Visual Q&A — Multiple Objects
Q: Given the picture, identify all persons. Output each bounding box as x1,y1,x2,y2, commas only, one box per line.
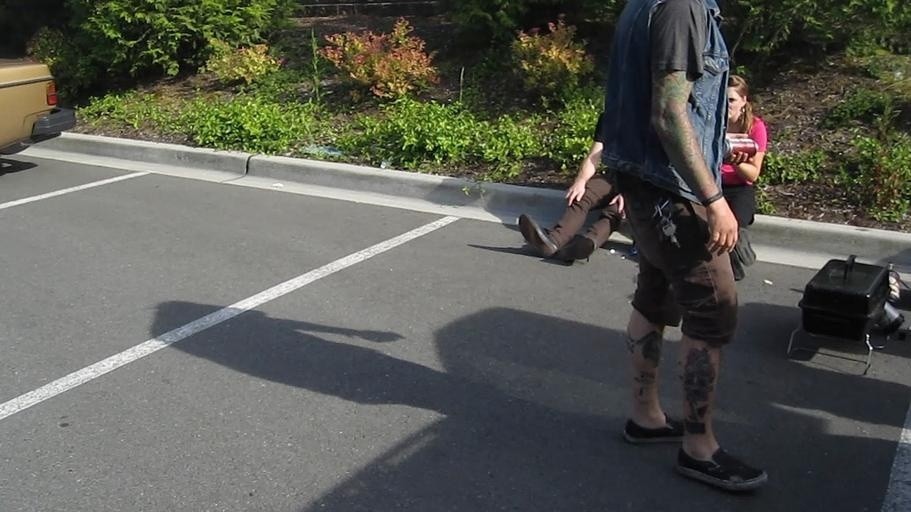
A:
597,0,774,494
518,111,629,264
715,74,771,282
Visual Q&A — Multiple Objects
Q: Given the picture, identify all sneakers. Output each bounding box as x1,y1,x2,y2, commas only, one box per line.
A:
677,446,767,491
518,214,559,259
623,413,683,443
556,235,597,264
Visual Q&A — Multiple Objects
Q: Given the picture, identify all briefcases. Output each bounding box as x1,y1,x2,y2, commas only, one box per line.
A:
798,254,892,340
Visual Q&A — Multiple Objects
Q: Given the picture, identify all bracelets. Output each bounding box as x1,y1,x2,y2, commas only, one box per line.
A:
700,192,725,207
